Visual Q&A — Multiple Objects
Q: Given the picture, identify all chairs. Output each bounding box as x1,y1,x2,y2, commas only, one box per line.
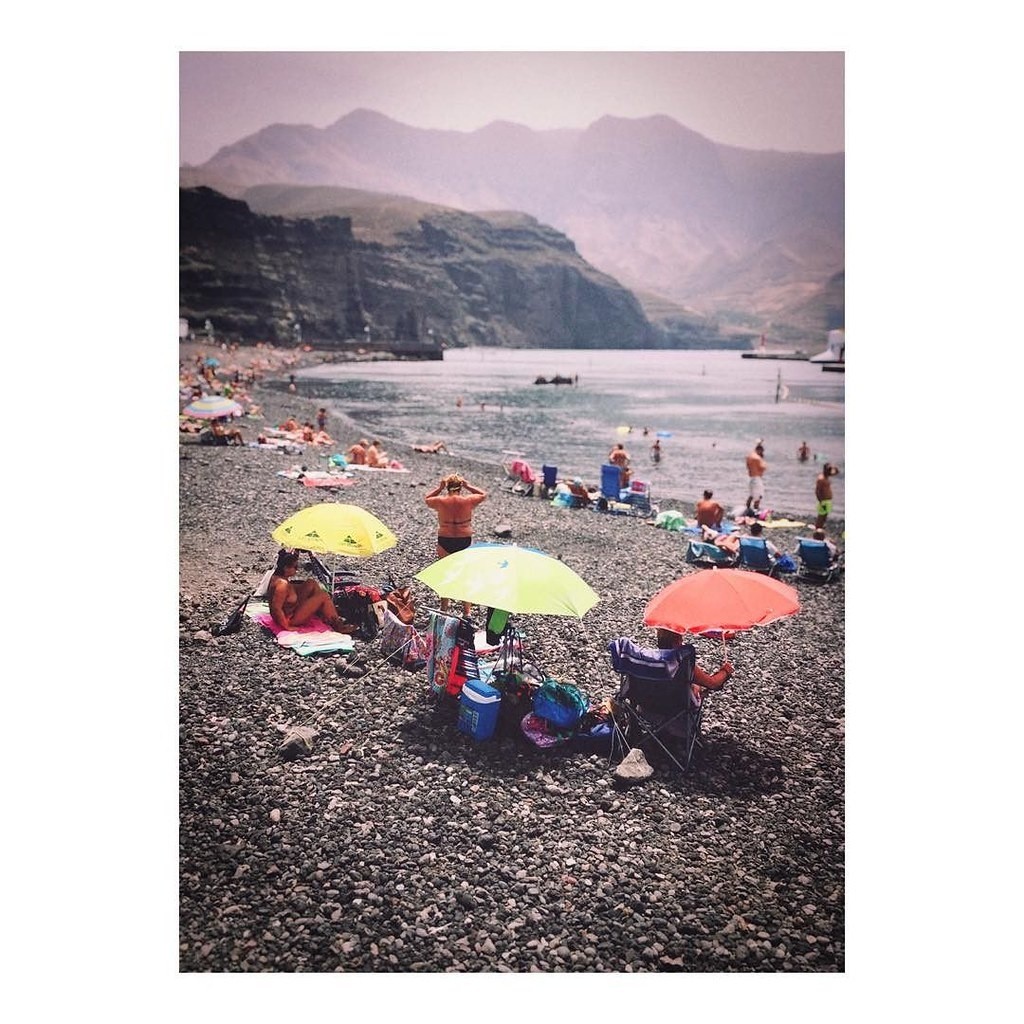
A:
734,535,788,579
495,451,664,520
306,551,360,593
605,633,707,775
794,535,845,583
688,539,734,570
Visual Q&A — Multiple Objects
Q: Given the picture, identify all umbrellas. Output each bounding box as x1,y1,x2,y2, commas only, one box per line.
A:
411,543,602,677
643,565,802,664
183,394,244,430
271,503,398,601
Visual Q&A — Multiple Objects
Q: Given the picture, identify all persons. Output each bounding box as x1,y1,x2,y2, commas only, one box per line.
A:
265,549,339,631
656,627,734,701
179,343,845,584
424,475,488,617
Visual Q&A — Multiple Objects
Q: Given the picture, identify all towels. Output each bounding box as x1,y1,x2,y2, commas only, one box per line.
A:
655,508,689,531
775,554,798,572
683,539,728,563
600,463,623,499
550,483,577,508
328,453,411,473
277,468,360,487
541,464,558,488
631,480,647,492
573,477,584,489
179,352,266,437
247,425,338,455
510,459,537,485
679,507,807,535
228,579,697,755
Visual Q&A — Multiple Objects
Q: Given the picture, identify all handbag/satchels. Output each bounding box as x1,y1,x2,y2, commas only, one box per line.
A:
255,569,277,598
211,595,252,635
384,577,413,624
534,679,591,727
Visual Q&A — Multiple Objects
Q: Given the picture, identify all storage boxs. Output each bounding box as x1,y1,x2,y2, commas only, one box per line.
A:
458,680,501,742
